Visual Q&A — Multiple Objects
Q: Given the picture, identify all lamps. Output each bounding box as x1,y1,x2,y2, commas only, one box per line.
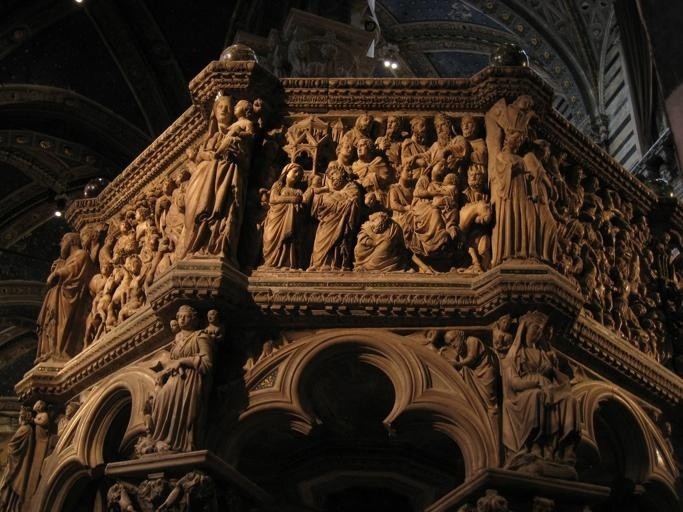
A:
53,198,65,218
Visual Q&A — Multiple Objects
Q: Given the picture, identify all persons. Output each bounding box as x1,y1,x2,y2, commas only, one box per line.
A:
32,141,200,364
486,95,682,376
436,309,582,481
1,400,52,511
151,305,224,452
184,95,498,273
